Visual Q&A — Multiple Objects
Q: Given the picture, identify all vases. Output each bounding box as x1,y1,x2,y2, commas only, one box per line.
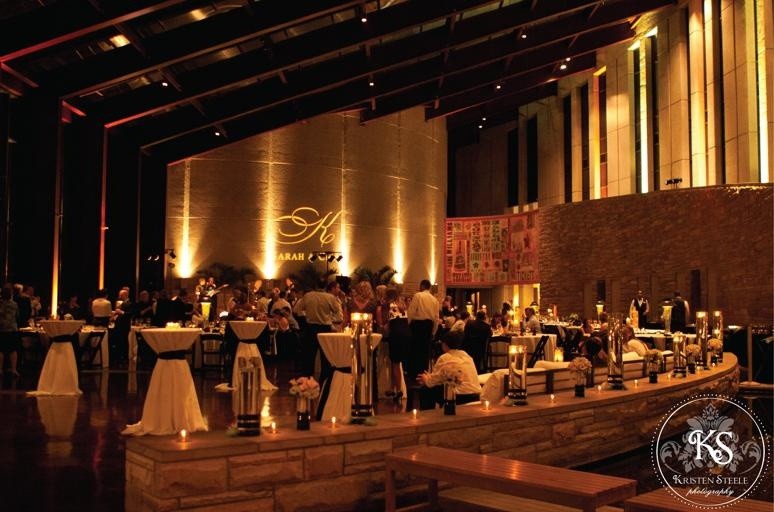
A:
710,349,719,365
686,356,698,373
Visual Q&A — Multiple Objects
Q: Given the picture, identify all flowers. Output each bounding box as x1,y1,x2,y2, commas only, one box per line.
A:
707,338,724,350
196,281,230,301
686,344,702,356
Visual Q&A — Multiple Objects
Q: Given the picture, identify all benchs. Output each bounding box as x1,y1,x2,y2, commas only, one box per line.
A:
384,443,637,512
622,486,774,511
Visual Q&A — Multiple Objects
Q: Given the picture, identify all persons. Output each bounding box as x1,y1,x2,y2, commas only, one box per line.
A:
1,275,690,411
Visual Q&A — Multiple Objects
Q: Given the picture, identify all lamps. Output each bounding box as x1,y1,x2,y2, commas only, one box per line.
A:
199,295,212,327
530,301,539,319
595,300,605,321
661,299,674,332
145,248,177,263
466,300,473,317
308,248,345,263
